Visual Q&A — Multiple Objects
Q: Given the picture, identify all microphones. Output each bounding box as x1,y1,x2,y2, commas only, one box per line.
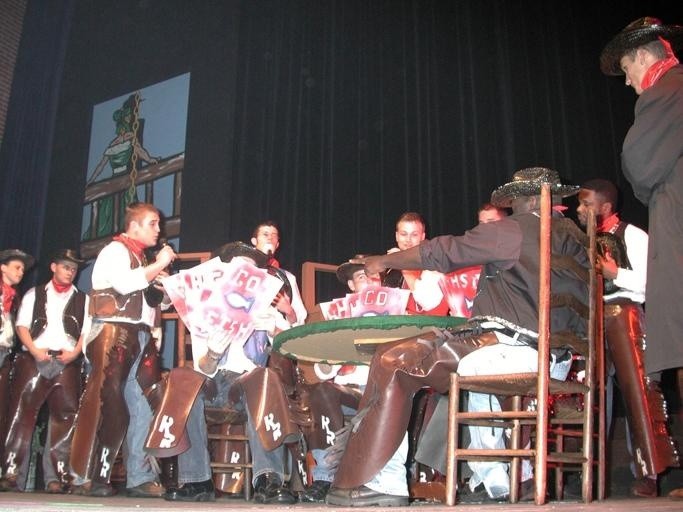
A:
159,238,173,269
268,250,272,267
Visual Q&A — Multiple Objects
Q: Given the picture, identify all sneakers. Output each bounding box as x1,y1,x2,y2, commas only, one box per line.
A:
634,477,658,498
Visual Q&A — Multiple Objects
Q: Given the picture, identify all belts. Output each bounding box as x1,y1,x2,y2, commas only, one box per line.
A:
483,319,552,361
47,349,63,356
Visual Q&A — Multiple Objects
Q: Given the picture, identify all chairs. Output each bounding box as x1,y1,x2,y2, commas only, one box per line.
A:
437,186,600,505
203,407,253,501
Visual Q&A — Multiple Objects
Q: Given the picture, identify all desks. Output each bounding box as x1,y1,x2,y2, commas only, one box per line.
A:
268,311,478,372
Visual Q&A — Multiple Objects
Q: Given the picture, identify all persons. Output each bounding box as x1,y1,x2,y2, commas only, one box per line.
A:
67,202,176,499
0,249,35,372
300,164,659,506
161,237,298,505
0,248,89,494
248,221,306,328
595,15,683,500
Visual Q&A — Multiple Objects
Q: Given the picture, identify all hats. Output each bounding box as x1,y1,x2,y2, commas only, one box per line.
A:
337,255,387,286
209,242,271,266
52,249,85,267
491,167,581,208
0,250,35,271
599,17,681,76
